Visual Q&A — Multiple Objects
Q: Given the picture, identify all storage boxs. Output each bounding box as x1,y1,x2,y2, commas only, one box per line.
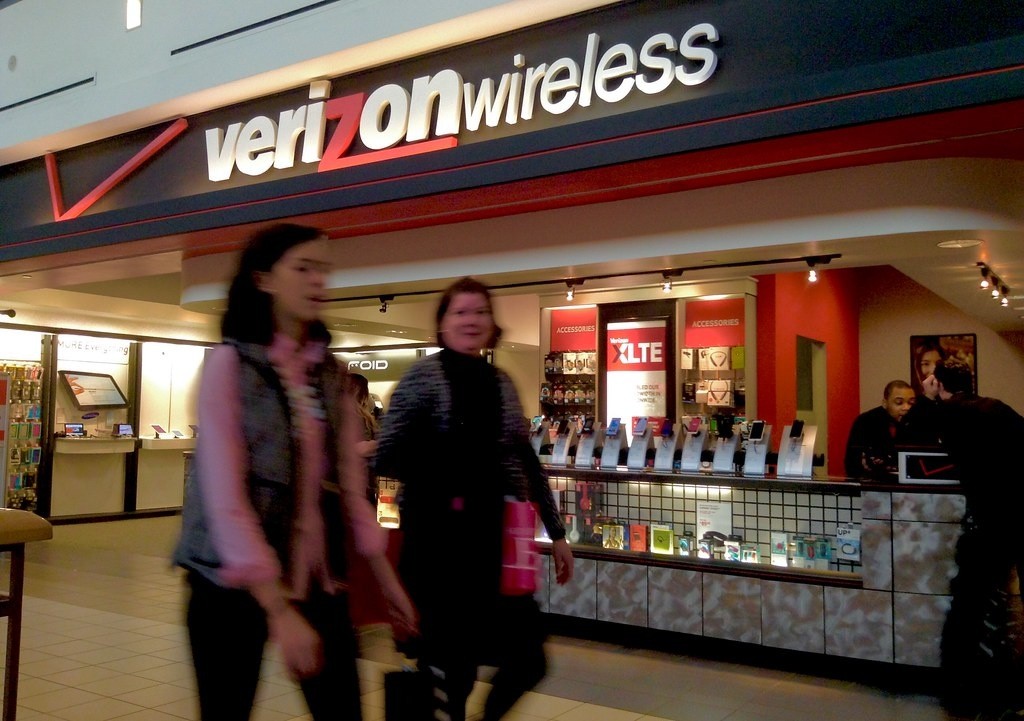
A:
708,347,730,371
708,378,734,408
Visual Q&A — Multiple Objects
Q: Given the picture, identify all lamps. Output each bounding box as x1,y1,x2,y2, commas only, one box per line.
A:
378,296,394,312
1001,285,1008,308
663,270,683,293
979,268,990,290
992,276,1000,300
564,279,585,301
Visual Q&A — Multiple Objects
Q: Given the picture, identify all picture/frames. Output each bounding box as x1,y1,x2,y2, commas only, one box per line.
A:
909,332,978,402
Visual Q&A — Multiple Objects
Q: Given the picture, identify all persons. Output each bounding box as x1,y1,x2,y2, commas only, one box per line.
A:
165,221,419,721
377,277,574,721
298,314,385,661
895,356,1023,621
65,374,84,395
842,378,915,482
910,340,943,396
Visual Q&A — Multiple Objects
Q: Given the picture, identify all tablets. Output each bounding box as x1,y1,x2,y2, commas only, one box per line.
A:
119,424,135,435
58,371,130,411
65,422,84,436
189,425,199,433
151,425,167,434
171,431,183,436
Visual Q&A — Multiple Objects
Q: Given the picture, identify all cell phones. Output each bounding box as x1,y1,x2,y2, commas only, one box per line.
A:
661,419,672,437
556,421,568,435
529,417,543,433
749,421,766,441
632,418,648,436
688,418,702,434
606,419,621,436
582,418,595,434
789,419,805,439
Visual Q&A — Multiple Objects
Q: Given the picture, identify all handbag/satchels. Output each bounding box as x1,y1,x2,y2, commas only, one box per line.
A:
383,626,452,721
500,471,544,598
